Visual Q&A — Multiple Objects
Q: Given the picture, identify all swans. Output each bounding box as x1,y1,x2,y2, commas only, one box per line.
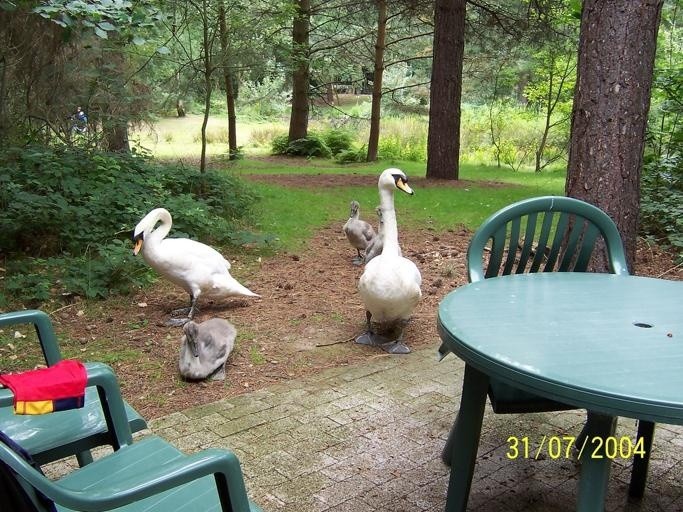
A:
355,167,422,354
342,200,376,262
132,208,262,327
352,206,384,266
178,317,238,382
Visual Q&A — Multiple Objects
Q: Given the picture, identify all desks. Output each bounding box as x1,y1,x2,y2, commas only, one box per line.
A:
432,272,682,511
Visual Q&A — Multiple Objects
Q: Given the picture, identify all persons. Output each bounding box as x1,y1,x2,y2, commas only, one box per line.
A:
68,106,86,135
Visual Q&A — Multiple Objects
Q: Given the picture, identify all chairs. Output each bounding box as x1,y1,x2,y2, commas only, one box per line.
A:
438,196,630,512
0,370,259,511
0,310,146,467
